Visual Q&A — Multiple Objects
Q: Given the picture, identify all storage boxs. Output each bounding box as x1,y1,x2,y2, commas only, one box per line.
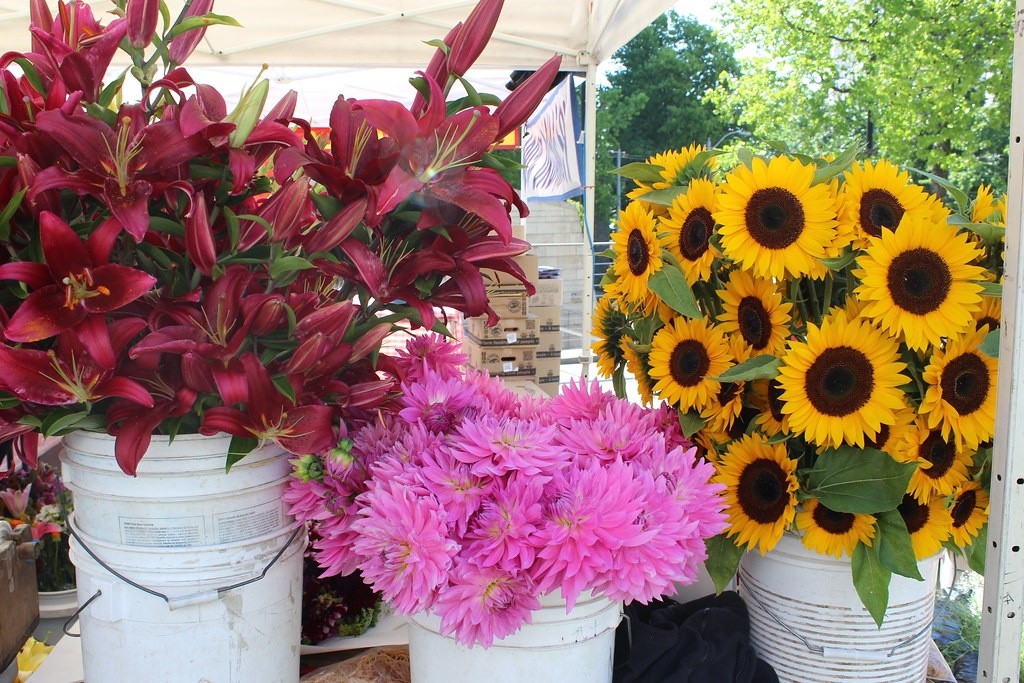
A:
459,222,564,401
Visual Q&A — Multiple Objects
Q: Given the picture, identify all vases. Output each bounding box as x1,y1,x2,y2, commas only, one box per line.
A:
56,431,303,683
732,518,950,683
405,577,623,683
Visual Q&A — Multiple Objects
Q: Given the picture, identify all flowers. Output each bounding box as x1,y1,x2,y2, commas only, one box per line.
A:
285,329,730,649
1,0,565,481
591,145,1013,629
